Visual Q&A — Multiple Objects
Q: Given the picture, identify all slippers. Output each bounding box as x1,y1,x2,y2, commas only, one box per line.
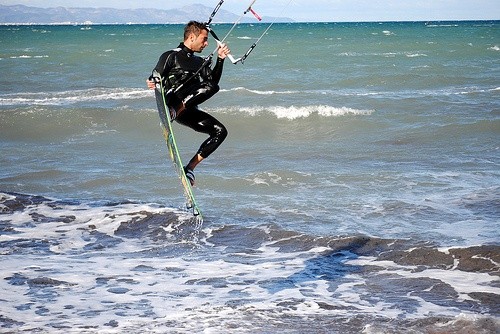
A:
183,164,196,187
166,106,178,123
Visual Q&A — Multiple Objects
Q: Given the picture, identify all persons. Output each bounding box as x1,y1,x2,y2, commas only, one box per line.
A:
145,19,232,189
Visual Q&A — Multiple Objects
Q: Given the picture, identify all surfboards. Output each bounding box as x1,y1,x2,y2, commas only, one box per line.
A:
151,68,200,219
